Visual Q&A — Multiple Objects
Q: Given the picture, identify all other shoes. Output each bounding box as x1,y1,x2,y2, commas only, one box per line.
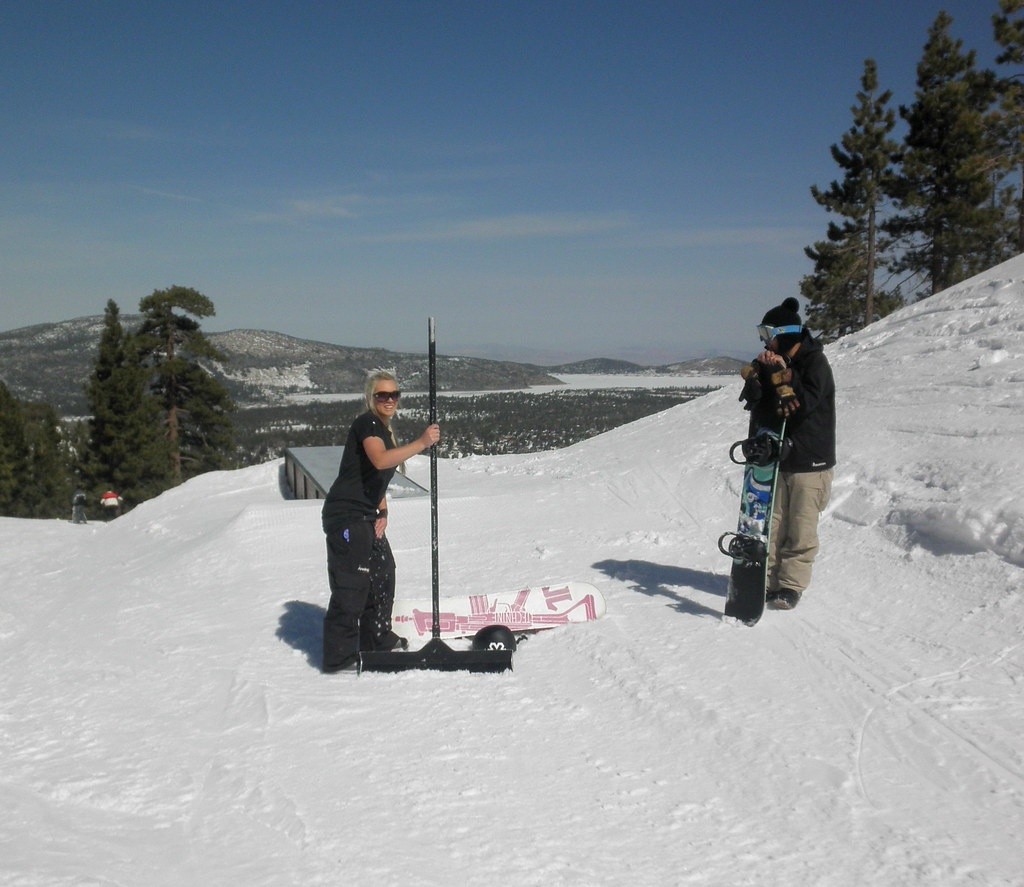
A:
772,588,799,609
392,637,409,652
335,661,358,673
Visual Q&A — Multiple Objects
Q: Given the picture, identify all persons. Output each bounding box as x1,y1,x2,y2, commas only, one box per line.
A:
318,370,442,675
750,295,837,612
71,484,88,524
100,487,124,522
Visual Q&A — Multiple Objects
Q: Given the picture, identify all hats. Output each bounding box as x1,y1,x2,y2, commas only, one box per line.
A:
760,297,802,351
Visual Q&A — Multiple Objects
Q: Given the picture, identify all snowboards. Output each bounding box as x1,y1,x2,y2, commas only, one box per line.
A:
716,364,796,627
391,580,606,644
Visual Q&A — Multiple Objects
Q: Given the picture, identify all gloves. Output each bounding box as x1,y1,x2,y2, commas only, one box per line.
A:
771,367,801,418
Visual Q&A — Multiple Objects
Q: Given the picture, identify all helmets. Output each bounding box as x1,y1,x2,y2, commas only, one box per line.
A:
471,625,517,652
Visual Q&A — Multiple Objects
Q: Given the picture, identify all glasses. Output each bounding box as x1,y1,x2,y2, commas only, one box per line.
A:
372,391,401,402
757,325,776,342
